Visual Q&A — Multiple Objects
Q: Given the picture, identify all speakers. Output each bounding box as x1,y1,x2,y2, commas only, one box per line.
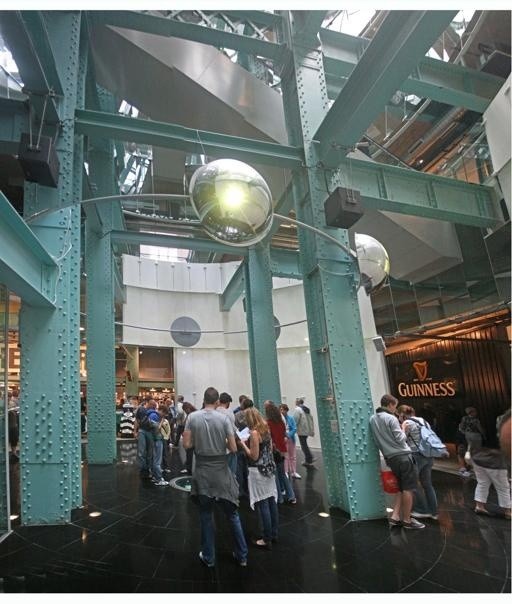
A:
324,186,364,229
18,132,61,189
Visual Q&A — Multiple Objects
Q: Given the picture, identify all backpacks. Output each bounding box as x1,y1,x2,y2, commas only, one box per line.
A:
140,414,152,432
407,417,449,458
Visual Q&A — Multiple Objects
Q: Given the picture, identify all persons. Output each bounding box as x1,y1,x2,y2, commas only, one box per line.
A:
237,407,282,550
471,408,511,519
202,393,317,503
370,394,426,529
0,390,19,413
117,394,198,485
458,406,487,475
397,404,448,519
183,387,249,566
80,405,88,434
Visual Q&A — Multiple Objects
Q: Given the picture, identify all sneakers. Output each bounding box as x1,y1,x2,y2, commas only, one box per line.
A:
302,458,317,466
180,470,188,474
505,511,511,520
389,510,438,530
251,537,273,550
232,551,247,566
279,472,301,505
199,551,214,569
148,468,171,485
474,508,496,517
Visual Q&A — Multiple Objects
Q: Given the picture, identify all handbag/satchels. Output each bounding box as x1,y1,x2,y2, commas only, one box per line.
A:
383,469,401,493
464,450,473,466
